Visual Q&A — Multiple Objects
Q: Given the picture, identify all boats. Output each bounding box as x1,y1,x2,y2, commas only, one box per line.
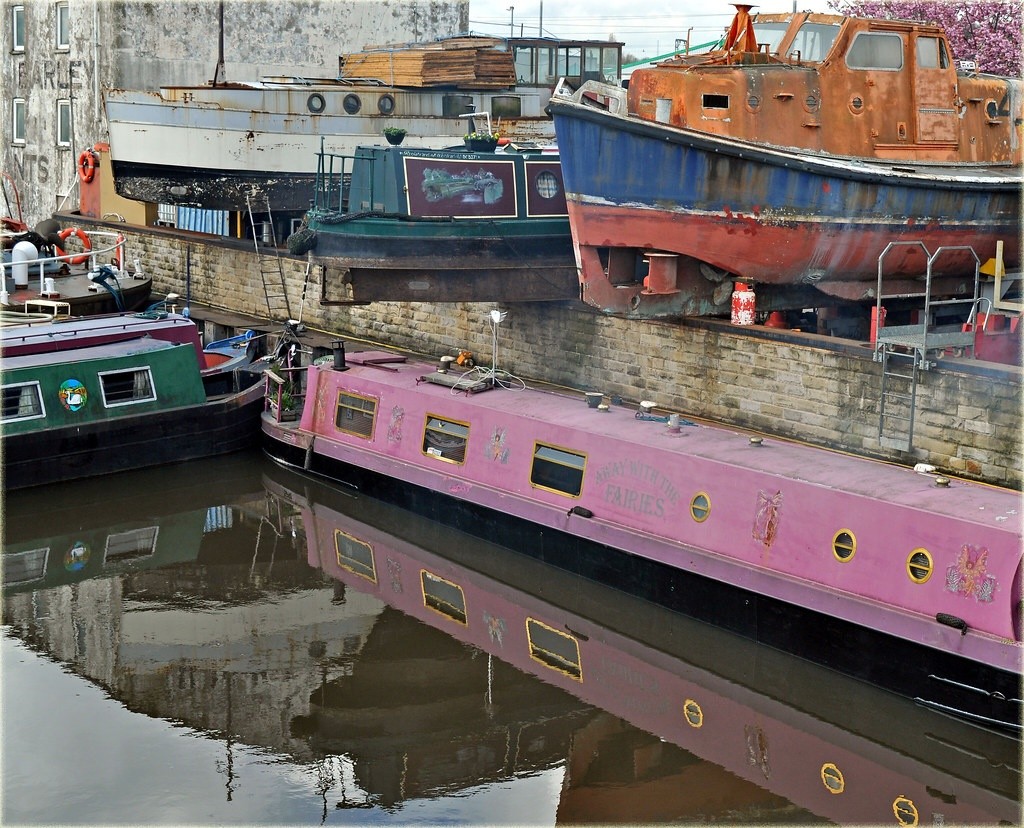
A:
269,475,1022,825
0,219,276,495
259,332,1023,679
98,32,626,218
540,1,1023,320
284,107,579,306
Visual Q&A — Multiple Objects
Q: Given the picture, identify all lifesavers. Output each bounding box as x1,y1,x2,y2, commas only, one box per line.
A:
56,227,91,267
76,150,96,184
